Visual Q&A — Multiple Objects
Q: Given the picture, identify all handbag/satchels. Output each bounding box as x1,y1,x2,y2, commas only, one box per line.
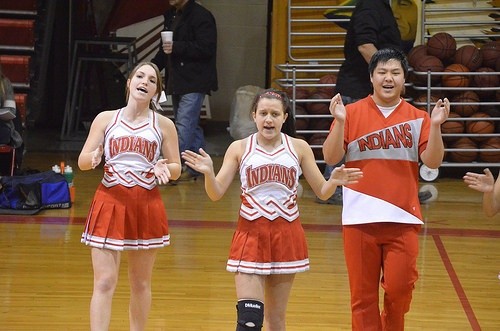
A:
0,171,70,214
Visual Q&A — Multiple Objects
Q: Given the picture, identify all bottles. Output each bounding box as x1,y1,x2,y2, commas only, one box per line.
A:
51,164,75,203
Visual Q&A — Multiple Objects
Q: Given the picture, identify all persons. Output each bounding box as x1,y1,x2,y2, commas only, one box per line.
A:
180,87,364,331
0,70,23,149
78,61,182,331
151,0,218,185
314,0,432,206
322,48,450,331
390,0,418,54
462,168,500,218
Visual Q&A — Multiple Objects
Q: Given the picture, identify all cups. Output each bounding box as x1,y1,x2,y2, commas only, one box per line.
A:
161,31,173,54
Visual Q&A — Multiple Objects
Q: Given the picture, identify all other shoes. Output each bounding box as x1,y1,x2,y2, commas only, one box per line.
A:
315,192,343,204
418,190,432,201
169,171,197,184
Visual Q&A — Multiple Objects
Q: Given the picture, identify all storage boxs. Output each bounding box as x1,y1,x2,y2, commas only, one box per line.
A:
69,185,77,204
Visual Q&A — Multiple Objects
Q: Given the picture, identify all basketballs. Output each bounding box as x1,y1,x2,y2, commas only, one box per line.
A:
283,32,500,162
